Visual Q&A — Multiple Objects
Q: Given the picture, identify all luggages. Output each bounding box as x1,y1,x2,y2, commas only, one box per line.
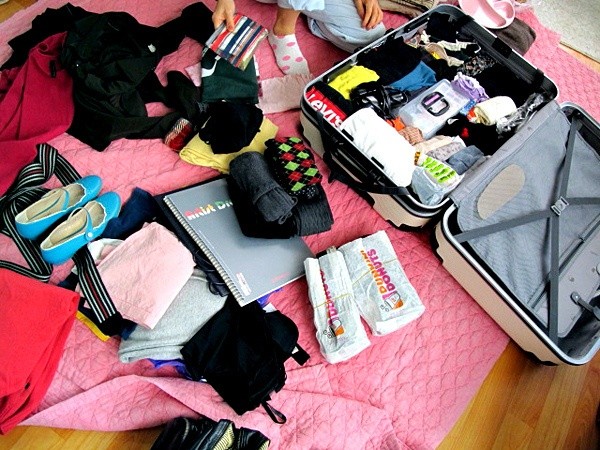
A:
300,3,600,364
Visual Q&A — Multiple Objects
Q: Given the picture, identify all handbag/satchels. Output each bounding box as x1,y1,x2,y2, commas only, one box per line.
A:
150,416,270,450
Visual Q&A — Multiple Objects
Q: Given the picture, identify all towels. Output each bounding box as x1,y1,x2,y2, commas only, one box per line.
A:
337,229,424,338
304,250,371,365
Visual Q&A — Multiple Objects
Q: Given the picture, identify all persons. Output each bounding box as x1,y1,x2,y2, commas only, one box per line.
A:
209,0,386,79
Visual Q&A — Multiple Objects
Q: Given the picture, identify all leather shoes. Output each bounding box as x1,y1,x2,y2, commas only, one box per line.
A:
14,175,102,240
39,192,121,265
